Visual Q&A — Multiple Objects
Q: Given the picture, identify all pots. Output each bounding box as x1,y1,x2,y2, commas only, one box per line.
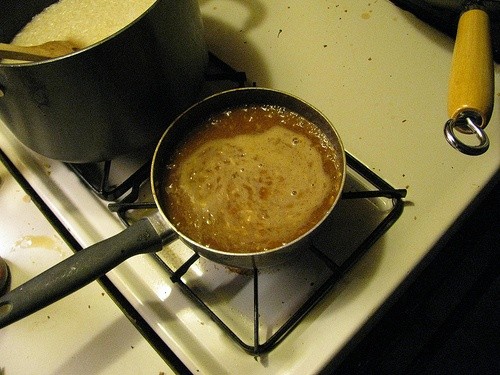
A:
0,0,209,165
430,0,499,155
0,88,347,329
